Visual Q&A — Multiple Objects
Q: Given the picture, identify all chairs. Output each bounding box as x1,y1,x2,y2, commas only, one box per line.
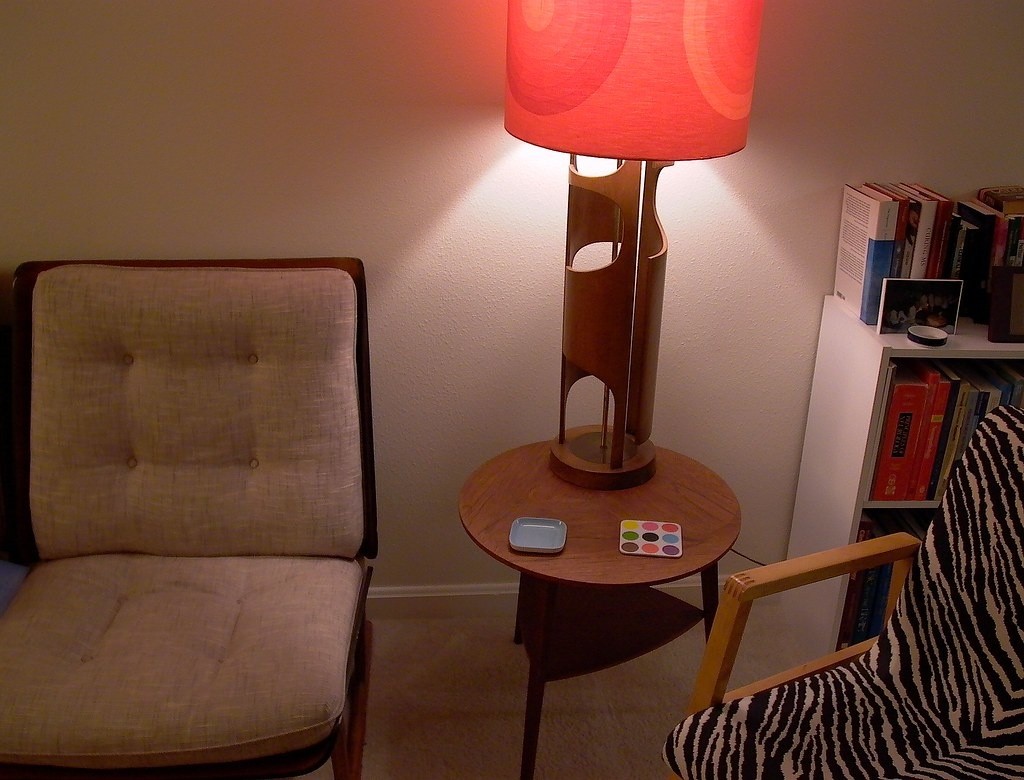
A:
0,257,376,780
661,404,1024,780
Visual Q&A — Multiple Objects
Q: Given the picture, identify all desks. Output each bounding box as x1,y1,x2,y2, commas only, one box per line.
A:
459,440,741,780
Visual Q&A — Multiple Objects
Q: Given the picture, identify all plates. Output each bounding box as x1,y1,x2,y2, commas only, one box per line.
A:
509,517,567,553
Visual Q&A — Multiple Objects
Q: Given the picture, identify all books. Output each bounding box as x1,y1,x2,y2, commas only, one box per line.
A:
837,510,929,648
869,359,1023,501
832,181,1023,344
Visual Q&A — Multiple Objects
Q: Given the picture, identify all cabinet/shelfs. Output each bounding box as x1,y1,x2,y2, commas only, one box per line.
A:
782,296,1024,658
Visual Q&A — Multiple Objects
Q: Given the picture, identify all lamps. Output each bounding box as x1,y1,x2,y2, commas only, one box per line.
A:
506,0,765,489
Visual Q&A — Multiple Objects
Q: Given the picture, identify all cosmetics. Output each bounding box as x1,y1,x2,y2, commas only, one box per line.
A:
620,519,682,556
